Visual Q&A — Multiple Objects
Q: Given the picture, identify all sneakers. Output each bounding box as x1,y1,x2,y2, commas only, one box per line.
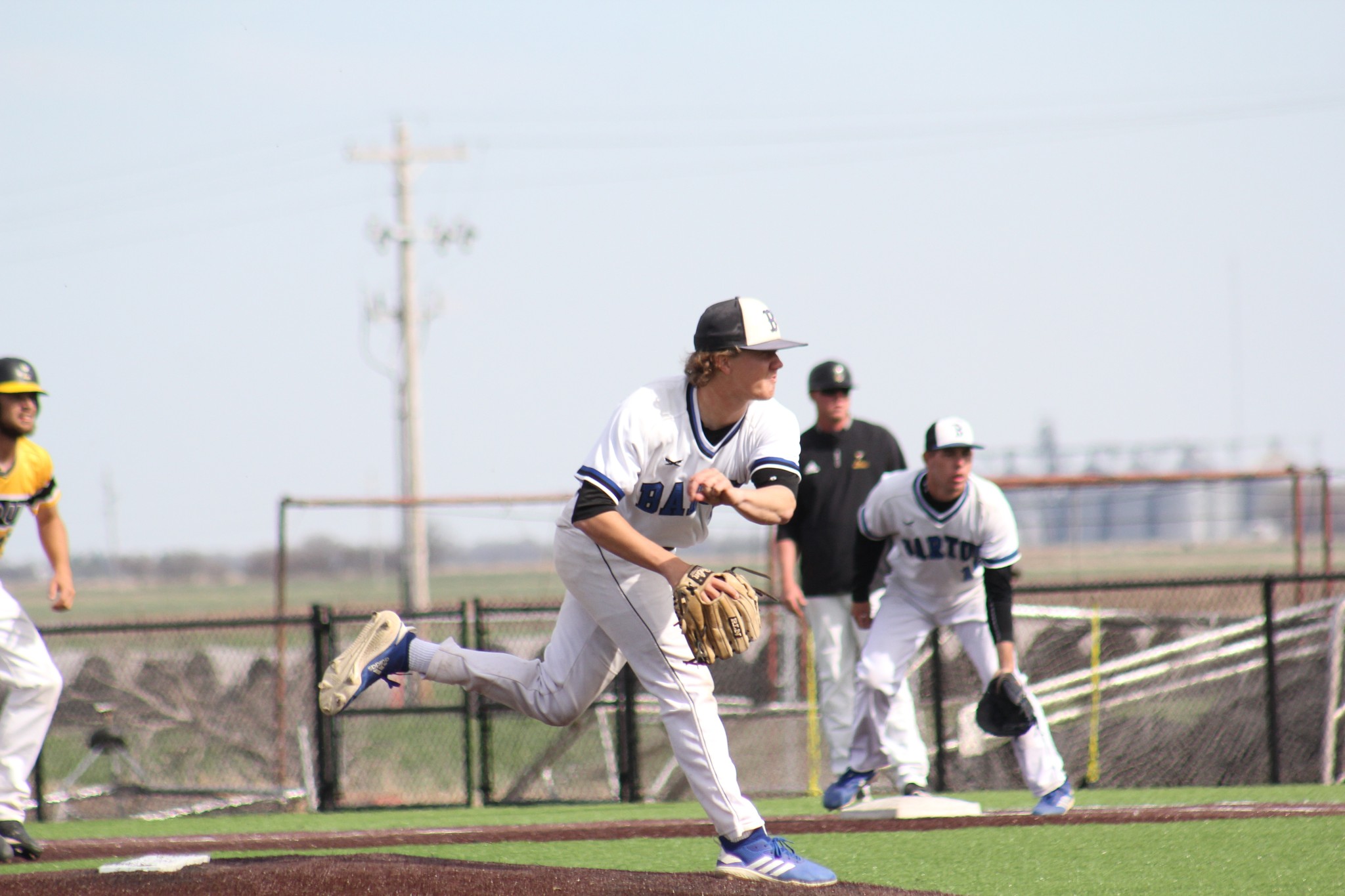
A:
823,767,879,811
1033,782,1076,816
716,829,838,888
318,610,416,716
0,821,43,861
903,784,930,797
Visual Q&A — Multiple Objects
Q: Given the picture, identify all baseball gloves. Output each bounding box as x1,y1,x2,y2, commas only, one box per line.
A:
672,564,762,664
975,672,1037,738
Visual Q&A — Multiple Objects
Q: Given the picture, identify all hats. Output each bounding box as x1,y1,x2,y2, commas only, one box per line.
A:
694,296,808,351
924,415,985,451
0,359,46,394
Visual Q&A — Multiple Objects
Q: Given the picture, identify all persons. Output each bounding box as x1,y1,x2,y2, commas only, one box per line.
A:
0,358,75,861
775,360,930,803
823,416,1075,817
318,297,840,886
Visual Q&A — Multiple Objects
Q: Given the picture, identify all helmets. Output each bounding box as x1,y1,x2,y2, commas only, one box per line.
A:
810,361,853,392
